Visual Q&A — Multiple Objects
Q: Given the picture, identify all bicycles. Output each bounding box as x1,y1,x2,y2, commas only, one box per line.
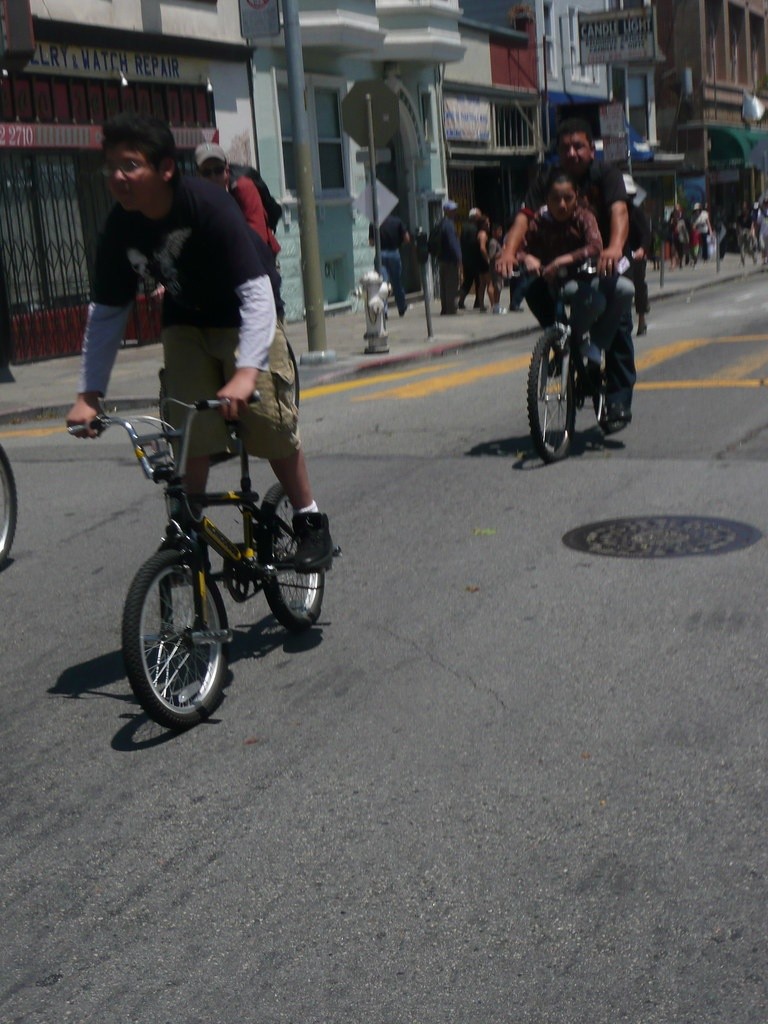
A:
0,444,17,569
69,396,343,729
508,263,627,462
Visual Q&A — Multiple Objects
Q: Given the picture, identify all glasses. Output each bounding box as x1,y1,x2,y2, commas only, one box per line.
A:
101,160,154,178
198,165,226,178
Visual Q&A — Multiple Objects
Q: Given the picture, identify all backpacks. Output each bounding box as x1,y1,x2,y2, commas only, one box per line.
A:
429,220,445,256
227,164,282,237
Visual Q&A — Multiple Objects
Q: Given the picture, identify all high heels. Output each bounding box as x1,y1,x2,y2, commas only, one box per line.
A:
637,326,647,335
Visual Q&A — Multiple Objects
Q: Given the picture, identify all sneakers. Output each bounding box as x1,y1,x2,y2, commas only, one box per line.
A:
294,511,335,574
174,550,211,572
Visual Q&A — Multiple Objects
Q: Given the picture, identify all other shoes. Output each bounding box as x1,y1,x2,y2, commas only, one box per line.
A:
510,305,524,312
473,302,478,307
605,387,632,433
459,302,465,309
493,305,505,314
399,305,407,317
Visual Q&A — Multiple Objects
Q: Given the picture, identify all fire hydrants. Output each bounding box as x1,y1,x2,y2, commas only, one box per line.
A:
351,271,393,354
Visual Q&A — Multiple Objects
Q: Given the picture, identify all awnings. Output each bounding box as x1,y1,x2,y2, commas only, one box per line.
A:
543,122,653,162
707,124,768,169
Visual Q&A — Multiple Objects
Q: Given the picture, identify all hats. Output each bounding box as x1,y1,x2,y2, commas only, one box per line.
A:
196,143,225,166
443,200,456,210
623,174,637,195
693,203,701,213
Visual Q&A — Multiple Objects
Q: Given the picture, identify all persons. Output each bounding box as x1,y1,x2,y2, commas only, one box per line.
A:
650,192,768,316
368,201,411,323
494,120,637,436
622,173,650,339
510,172,606,376
67,114,334,576
431,201,504,319
192,141,284,325
